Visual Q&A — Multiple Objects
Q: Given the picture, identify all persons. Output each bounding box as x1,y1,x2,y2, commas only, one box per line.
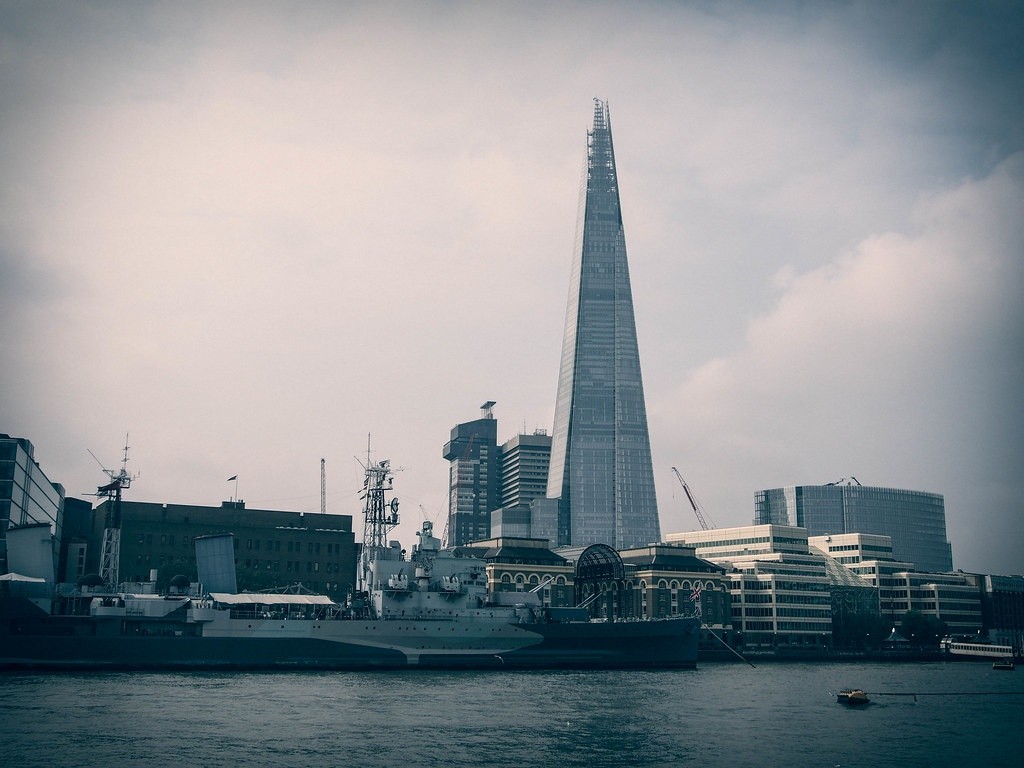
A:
259,605,331,621
840,687,866,696
131,625,199,639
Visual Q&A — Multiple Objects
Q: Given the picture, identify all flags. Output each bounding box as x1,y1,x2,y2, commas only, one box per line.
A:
690,587,701,599
227,475,236,481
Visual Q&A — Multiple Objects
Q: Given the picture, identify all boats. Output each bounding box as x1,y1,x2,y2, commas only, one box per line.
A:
939,642,1024,663
0,457,701,674
837,690,870,706
